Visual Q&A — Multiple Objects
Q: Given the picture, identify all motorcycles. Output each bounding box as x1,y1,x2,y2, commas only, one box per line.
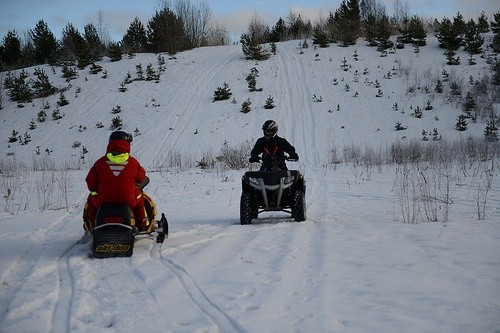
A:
82,176,169,258
240,156,308,225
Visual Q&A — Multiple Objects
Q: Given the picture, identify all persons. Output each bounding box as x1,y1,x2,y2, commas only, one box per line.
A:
248,119,300,170
86,130,147,232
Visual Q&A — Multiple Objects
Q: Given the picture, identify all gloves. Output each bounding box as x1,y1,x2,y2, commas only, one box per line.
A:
251,156,261,163
290,153,299,161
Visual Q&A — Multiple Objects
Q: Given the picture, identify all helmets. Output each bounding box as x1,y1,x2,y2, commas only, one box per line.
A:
261,119,280,139
108,130,132,144
